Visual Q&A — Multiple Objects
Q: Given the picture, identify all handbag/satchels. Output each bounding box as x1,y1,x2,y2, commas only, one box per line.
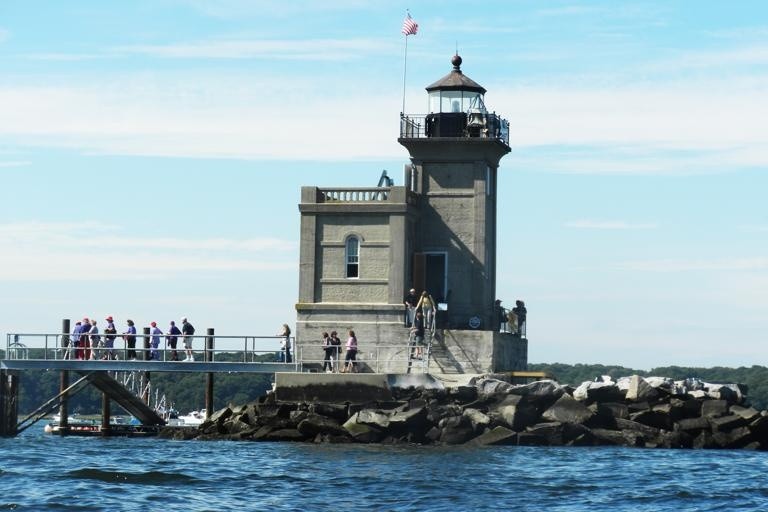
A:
324,340,332,350
104,329,116,339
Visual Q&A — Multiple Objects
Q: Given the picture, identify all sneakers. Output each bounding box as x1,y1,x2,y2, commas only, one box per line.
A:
182,358,194,361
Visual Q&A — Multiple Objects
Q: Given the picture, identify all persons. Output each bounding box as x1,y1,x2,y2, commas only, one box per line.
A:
341,330,359,373
404,288,419,326
330,331,342,373
321,332,336,373
72,315,196,362
495,299,528,335
415,290,436,329
410,312,425,358
276,323,292,363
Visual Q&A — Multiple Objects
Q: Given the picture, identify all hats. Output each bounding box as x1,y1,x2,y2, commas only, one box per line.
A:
76,318,96,324
151,322,156,327
106,317,112,320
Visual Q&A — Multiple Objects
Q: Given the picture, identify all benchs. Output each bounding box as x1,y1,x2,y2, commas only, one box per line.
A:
511,372,546,385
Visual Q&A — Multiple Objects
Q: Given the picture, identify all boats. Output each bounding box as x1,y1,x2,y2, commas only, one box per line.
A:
113,416,126,423
53,414,83,421
178,409,206,426
